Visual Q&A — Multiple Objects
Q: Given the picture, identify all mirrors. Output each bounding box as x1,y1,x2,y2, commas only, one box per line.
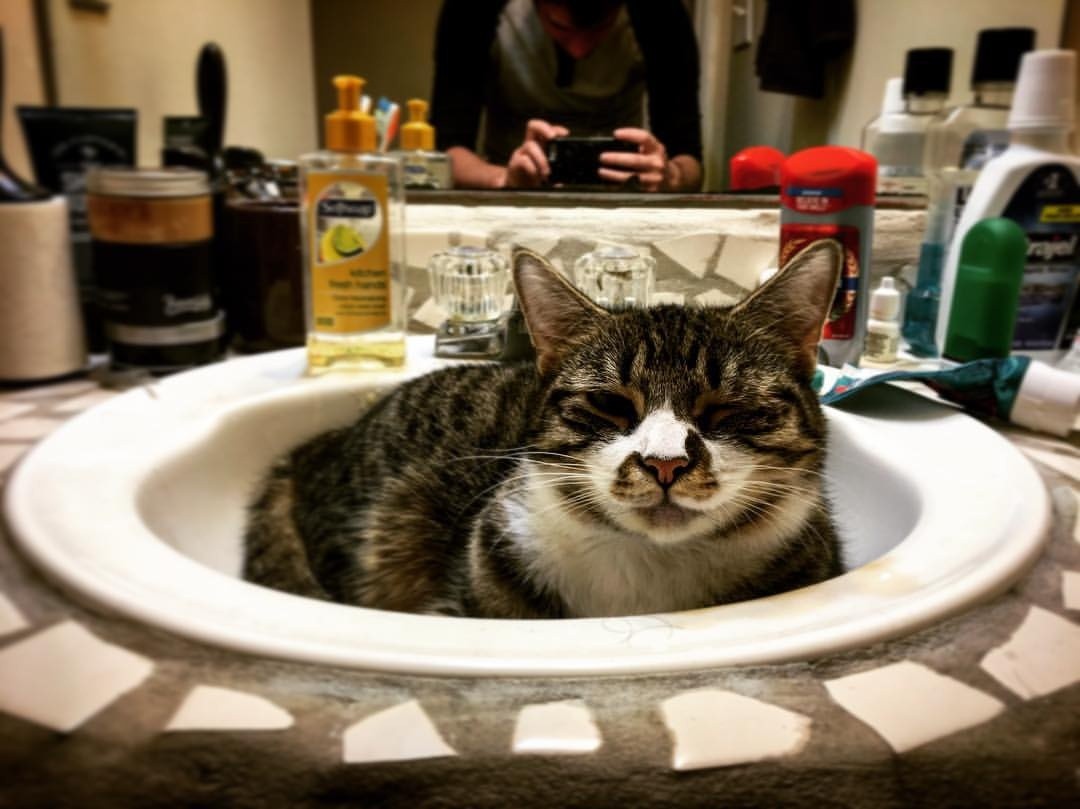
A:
48,5,1079,206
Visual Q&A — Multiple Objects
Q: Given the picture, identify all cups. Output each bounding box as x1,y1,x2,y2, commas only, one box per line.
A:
227,198,305,354
87,168,230,376
1,196,95,387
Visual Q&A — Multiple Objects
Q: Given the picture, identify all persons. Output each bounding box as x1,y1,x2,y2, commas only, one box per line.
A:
431,0,705,194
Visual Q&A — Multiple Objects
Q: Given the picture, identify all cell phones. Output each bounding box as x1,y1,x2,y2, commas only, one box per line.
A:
541,136,644,183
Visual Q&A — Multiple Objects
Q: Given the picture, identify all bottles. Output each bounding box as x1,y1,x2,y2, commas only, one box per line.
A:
926,26,1039,280
778,146,876,367
936,49,1080,370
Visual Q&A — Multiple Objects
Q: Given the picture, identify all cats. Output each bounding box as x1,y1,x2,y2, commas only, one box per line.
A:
246,238,854,623
